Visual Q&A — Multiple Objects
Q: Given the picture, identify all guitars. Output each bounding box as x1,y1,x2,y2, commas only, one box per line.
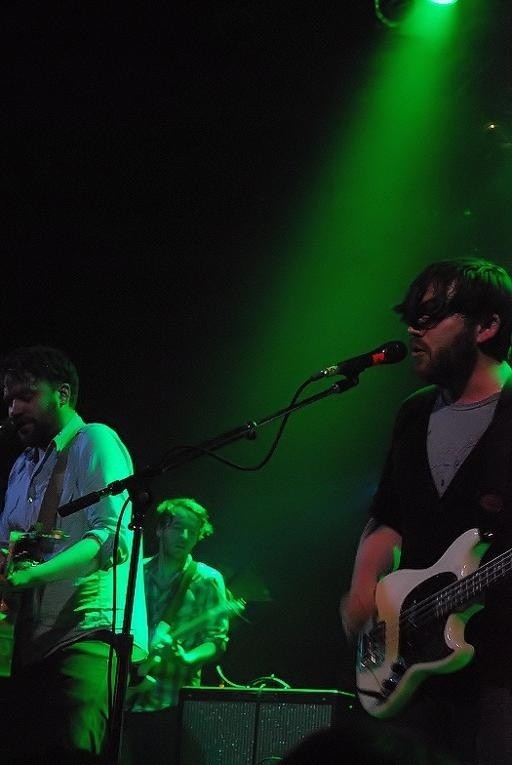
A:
1,529,69,682
355,525,512,721
125,598,246,705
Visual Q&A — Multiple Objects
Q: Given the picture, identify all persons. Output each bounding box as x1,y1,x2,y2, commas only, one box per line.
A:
341,259,512,765
118,497,229,765
0,345,149,765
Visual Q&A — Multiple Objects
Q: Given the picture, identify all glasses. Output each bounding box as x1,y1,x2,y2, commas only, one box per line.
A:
397,296,461,331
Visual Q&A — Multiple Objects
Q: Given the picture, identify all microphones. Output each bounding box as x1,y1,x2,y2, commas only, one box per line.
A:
320,341,407,378
